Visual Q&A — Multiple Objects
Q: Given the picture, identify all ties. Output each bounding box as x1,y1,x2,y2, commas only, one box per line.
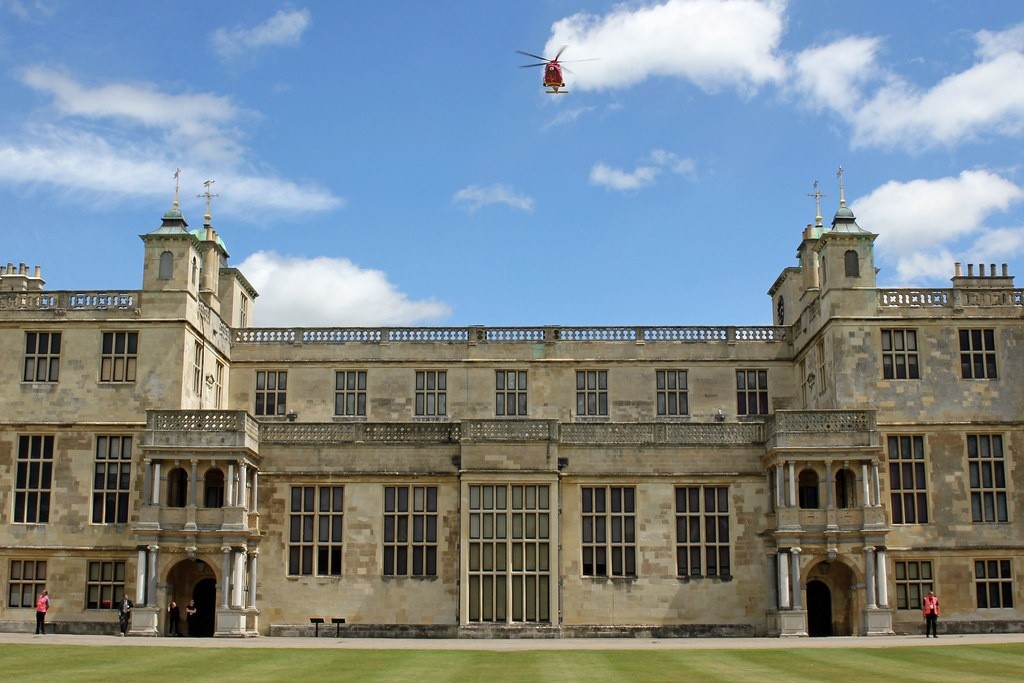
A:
124,600,127,613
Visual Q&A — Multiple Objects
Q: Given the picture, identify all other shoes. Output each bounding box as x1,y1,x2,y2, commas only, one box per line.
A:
35,632,40,634
175,634,178,636
169,634,172,636
119,632,124,636
926,634,929,638
933,635,938,638
42,632,46,634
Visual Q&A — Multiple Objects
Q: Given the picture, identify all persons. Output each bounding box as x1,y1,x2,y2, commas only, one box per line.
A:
923,591,940,638
118,594,134,636
34,589,50,634
186,600,197,637
167,601,180,637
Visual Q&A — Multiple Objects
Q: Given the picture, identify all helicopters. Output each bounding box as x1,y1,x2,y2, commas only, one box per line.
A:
516,45,601,93
